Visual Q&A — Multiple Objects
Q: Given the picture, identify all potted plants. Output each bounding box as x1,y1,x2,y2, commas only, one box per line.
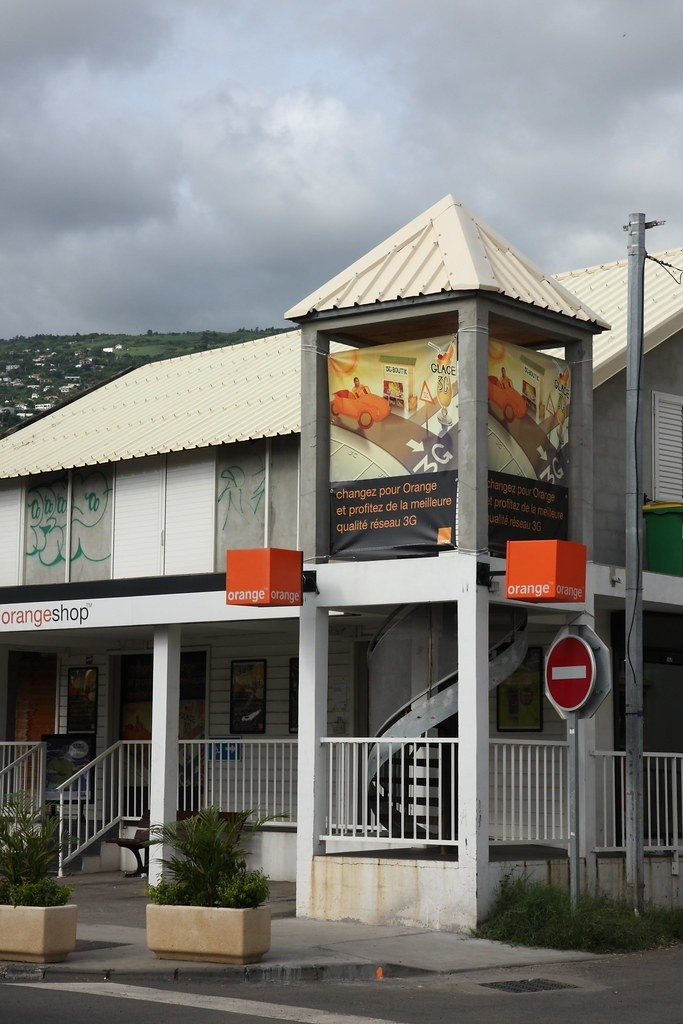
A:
139,805,292,964
0,789,78,964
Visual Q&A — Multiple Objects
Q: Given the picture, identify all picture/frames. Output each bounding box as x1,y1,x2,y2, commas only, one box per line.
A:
496,646,543,732
289,657,299,733
67,666,99,734
230,659,267,734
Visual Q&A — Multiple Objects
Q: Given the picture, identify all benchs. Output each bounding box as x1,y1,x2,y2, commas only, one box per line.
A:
106,810,248,878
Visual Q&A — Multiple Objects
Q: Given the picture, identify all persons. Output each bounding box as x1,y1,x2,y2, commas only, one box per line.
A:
352,377,368,398
499,367,511,390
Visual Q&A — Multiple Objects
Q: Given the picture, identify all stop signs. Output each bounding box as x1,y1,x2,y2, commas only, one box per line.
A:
545,635,594,711
